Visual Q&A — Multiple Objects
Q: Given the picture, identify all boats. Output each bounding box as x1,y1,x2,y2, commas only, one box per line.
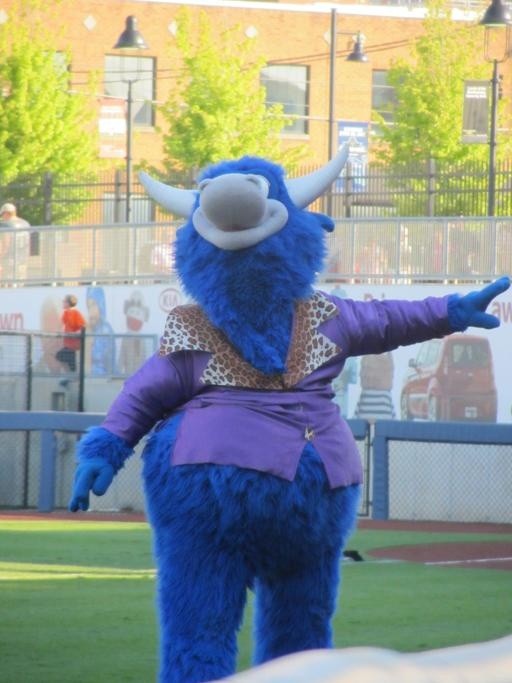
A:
338,127,367,154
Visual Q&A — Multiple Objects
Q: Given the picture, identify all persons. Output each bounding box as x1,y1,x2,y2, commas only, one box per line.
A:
55,292,85,376
0,202,31,230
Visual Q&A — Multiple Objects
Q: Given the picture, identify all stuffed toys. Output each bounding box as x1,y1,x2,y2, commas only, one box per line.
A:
69,140,511,683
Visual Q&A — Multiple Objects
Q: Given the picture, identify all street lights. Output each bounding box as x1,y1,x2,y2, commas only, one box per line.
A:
478,1,511,216
112,16,150,223
327,8,369,218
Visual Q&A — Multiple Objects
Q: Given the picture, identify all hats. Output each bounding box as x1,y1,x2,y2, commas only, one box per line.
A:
0,203,16,216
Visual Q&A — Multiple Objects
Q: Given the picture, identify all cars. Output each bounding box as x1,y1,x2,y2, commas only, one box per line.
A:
400,333,499,425
